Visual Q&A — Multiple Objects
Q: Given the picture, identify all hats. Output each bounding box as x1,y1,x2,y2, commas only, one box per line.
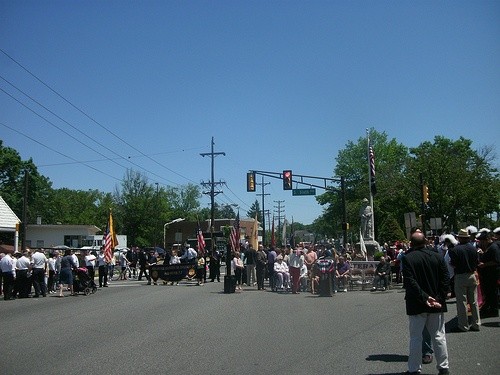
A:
457,228,471,238
466,225,478,234
493,227,500,233
478,227,491,233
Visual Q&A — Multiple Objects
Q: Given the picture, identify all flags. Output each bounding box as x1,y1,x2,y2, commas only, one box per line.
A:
102,214,118,263
250,216,258,251
229,213,240,253
196,216,206,252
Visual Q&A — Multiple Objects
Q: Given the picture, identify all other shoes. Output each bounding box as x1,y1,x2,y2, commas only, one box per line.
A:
370,286,376,292
381,287,386,291
103,285,108,287
48,290,56,295
71,293,77,296
407,370,420,375
3,296,15,301
99,285,102,287
468,326,480,331
33,294,39,298
210,280,214,282
422,353,433,364
343,288,347,292
235,286,242,291
258,287,266,291
437,368,449,375
449,326,466,332
147,282,157,285
59,294,65,296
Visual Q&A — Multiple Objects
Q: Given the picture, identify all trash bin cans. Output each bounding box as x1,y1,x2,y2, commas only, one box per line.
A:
224,275,236,293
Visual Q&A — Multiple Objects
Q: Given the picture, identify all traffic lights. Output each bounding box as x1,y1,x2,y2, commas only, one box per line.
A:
422,185,429,203
283,169,292,190
342,223,349,230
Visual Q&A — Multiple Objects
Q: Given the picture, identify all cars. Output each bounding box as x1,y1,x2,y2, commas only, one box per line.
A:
144,246,165,257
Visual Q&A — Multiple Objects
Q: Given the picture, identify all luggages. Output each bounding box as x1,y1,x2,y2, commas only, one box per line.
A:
223,267,236,293
319,273,336,297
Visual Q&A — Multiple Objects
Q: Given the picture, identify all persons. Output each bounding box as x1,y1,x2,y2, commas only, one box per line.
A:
466,231,500,318
0,247,110,301
281,243,337,297
336,245,375,269
274,254,290,289
427,237,456,299
233,241,256,290
335,257,350,292
121,244,221,285
256,244,268,291
372,240,411,291
449,229,480,332
360,198,372,239
263,243,280,291
402,232,450,375
400,227,439,363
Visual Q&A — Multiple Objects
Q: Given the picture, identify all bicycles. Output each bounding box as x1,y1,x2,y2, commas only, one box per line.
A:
109,262,138,281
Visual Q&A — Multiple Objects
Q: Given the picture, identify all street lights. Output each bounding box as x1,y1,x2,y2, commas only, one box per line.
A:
401,176,427,237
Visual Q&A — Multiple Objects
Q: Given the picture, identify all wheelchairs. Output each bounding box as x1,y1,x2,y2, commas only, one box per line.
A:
269,270,293,292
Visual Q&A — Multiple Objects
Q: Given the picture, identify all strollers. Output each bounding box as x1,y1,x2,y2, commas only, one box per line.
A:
72,267,97,296
